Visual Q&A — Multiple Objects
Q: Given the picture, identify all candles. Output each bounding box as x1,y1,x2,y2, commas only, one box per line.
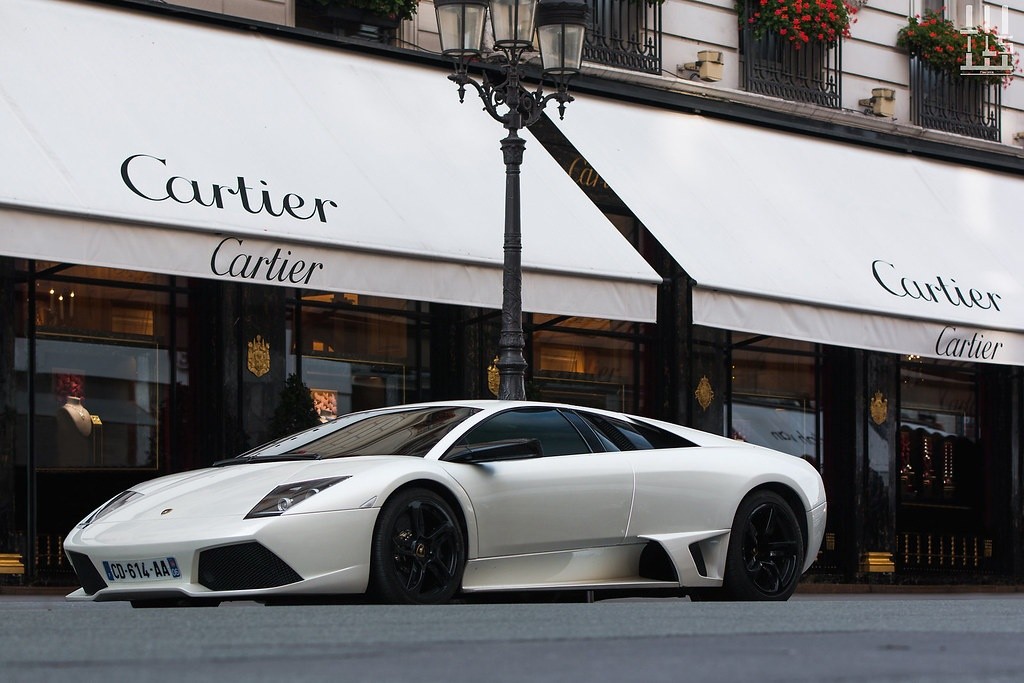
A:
58,296,64,319
50,289,54,307
69,292,75,318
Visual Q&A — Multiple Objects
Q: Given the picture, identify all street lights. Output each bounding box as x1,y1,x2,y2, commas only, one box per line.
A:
431,0,591,403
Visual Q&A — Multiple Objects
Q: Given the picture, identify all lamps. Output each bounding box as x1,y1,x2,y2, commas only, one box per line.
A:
859,88,896,118
684,50,725,81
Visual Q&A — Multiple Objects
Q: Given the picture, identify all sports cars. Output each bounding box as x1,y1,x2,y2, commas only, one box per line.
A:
61,395,830,609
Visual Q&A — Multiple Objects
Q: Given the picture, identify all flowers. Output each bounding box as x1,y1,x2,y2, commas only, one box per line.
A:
737,0,869,49
317,0,421,24
899,7,1022,88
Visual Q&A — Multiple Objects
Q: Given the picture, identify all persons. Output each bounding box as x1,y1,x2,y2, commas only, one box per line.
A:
48,396,96,486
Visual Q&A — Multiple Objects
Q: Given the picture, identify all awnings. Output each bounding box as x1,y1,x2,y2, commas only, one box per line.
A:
523,80,1024,364
0,0,669,323
724,402,890,488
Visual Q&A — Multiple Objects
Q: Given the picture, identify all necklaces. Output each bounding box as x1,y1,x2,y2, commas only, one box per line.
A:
67,405,85,419
